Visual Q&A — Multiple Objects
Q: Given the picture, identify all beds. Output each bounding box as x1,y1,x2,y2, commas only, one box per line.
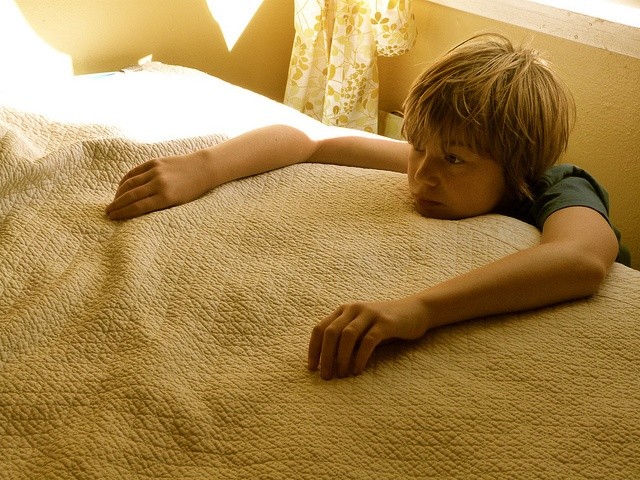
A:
0,56,640,480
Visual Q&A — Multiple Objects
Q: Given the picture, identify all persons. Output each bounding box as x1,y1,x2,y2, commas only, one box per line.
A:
106,33,632,381
